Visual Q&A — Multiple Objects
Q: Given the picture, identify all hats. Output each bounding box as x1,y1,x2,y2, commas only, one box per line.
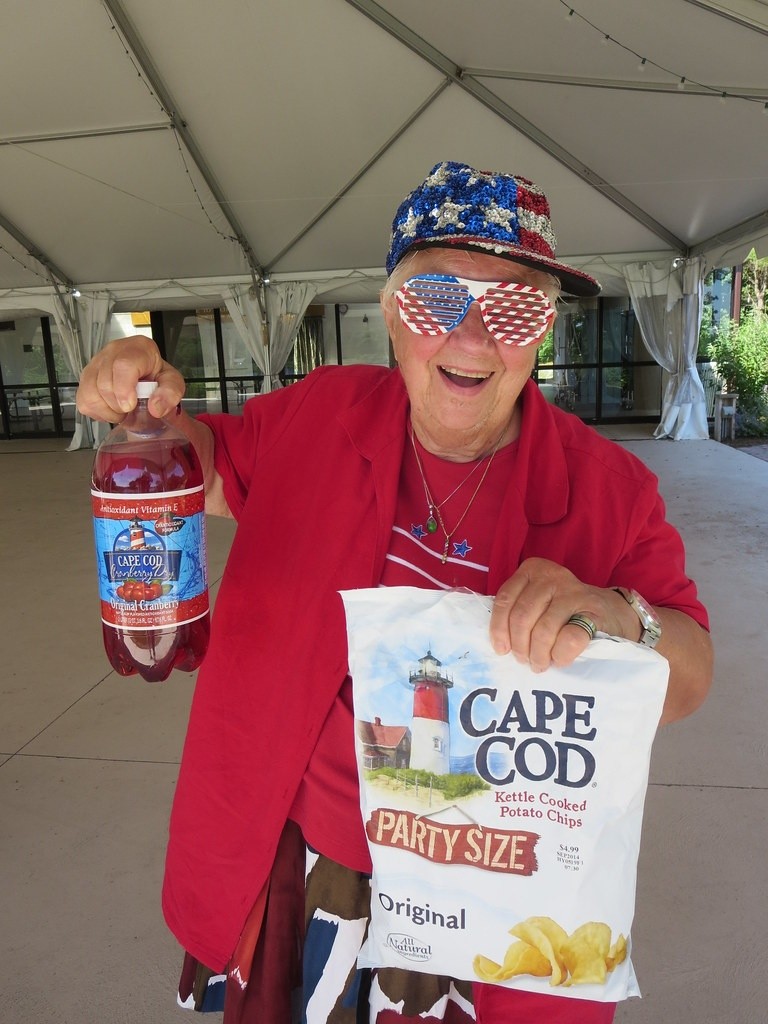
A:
386,162,601,297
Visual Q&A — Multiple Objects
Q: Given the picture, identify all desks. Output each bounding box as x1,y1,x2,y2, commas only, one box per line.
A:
4,387,51,432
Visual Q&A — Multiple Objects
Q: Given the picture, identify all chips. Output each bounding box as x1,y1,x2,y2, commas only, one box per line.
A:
473,915,631,986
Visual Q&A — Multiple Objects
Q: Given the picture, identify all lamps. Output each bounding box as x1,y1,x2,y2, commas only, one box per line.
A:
263,271,270,282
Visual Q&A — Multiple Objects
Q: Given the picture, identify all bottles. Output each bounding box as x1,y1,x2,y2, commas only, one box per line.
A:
89,381,210,683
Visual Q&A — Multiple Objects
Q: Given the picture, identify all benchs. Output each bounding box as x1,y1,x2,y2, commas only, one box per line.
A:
7,396,27,421
23,395,64,420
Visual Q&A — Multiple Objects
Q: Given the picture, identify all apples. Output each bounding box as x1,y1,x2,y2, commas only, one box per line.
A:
116,581,162,602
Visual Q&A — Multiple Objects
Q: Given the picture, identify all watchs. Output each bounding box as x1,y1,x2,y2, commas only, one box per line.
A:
609,583,662,652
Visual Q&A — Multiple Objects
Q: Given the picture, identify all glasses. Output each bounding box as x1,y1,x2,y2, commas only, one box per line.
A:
391,274,557,347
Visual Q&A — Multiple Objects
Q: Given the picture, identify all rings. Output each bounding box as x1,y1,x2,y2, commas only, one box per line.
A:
567,613,598,640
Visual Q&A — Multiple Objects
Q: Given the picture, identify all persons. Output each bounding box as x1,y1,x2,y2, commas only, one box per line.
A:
71,162,714,1023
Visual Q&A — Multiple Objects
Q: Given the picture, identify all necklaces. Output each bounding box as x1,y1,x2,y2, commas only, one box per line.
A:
406,417,524,567
